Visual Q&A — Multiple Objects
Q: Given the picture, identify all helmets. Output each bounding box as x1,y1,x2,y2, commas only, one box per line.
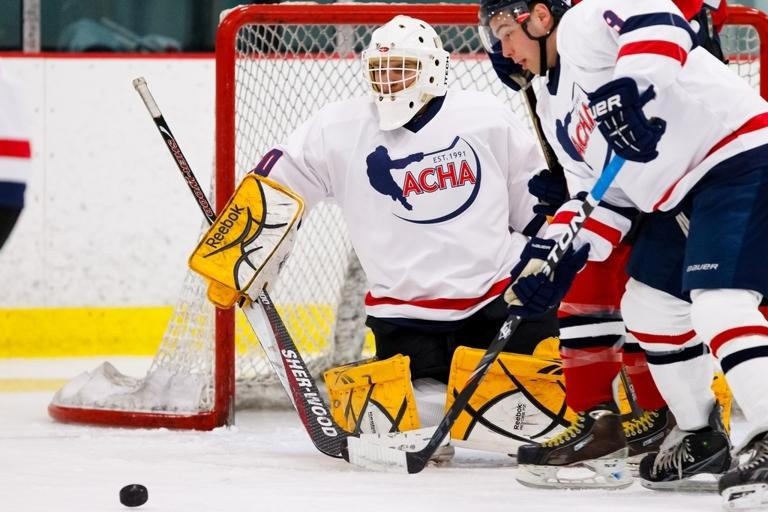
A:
362,14,460,132
477,0,572,92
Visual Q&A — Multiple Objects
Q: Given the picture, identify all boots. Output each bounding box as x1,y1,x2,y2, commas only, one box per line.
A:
640,399,730,484
718,430,768,497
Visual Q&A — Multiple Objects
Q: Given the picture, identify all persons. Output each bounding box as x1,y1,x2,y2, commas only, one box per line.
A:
186,15,575,448
479,0,767,499
479,20,669,468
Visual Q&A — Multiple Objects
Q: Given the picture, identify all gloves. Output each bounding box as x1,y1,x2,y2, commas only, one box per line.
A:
527,163,566,217
500,237,590,319
589,78,666,162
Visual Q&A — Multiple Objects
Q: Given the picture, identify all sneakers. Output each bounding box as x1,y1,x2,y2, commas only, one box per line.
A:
624,406,677,465
518,398,630,468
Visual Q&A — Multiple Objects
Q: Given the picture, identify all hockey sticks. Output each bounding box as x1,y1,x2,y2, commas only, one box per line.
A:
340,154,625,473
133,77,452,458
